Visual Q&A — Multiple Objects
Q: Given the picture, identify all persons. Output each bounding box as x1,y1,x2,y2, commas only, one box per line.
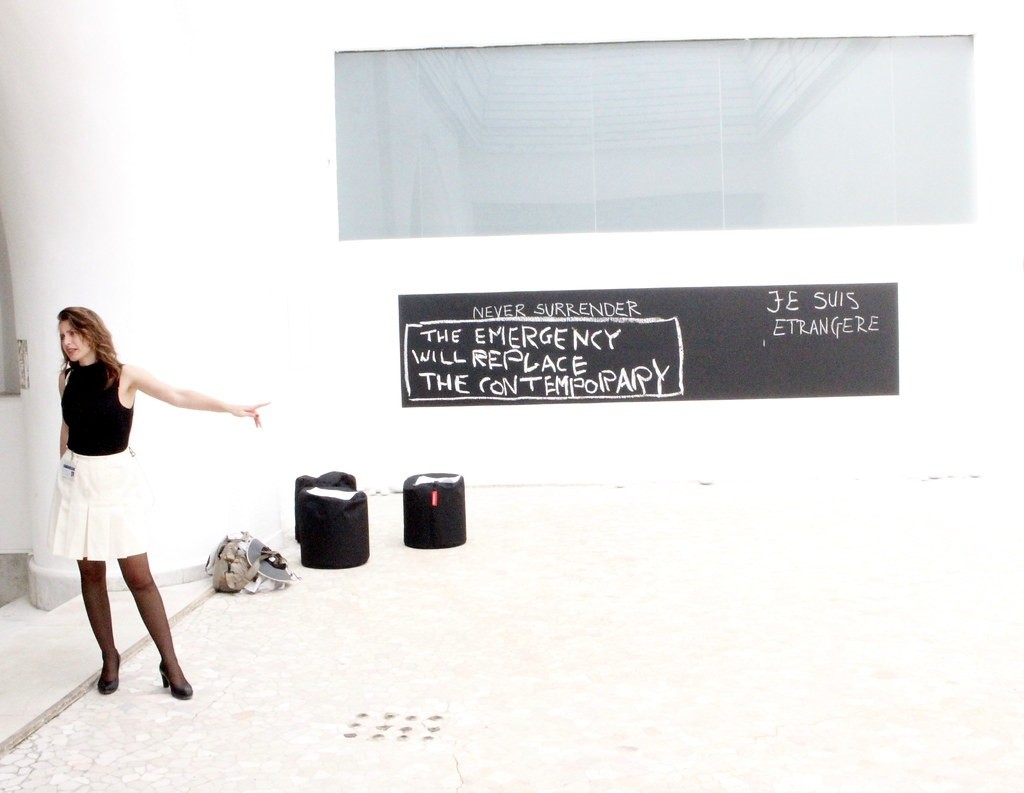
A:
46,306,271,700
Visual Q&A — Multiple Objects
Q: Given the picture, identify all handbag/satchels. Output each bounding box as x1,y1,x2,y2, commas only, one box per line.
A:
205,532,254,592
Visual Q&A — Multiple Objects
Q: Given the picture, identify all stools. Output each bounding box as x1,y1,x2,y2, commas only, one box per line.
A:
402,475,468,549
298,488,372,571
293,471,358,548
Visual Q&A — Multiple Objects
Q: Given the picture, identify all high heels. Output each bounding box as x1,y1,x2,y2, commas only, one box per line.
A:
98,652,120,695
160,660,193,698
